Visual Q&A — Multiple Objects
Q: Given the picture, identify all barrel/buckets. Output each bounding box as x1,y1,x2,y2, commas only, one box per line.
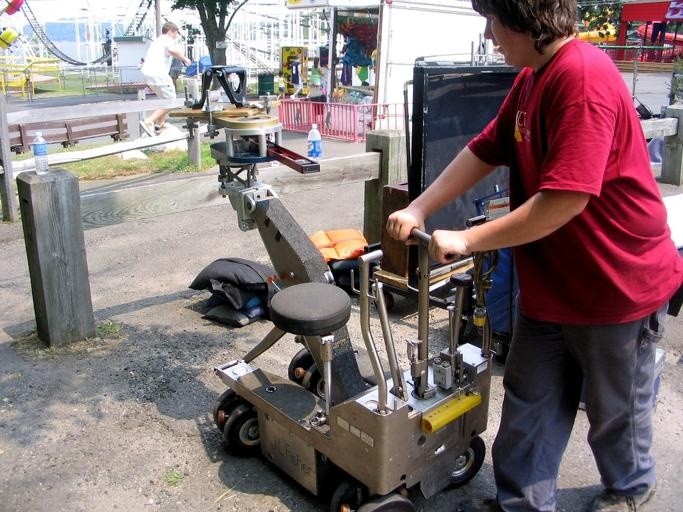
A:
258,73,274,96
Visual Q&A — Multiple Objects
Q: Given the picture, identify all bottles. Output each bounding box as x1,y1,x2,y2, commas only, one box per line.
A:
307,124,323,163
33,130,49,176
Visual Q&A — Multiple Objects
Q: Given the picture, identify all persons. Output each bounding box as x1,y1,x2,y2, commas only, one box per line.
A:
287,54,305,100
381,1,682,512
137,20,191,138
275,70,290,102
306,57,325,99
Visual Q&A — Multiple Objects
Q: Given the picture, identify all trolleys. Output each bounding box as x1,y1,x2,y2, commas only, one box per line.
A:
211,177,496,510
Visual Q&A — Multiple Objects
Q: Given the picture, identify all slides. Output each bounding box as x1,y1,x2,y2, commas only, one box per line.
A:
572,5,618,43
620,0,682,63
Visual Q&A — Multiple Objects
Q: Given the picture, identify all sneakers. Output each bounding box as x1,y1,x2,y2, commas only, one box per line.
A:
587,483,658,512
154,123,169,133
138,117,155,138
